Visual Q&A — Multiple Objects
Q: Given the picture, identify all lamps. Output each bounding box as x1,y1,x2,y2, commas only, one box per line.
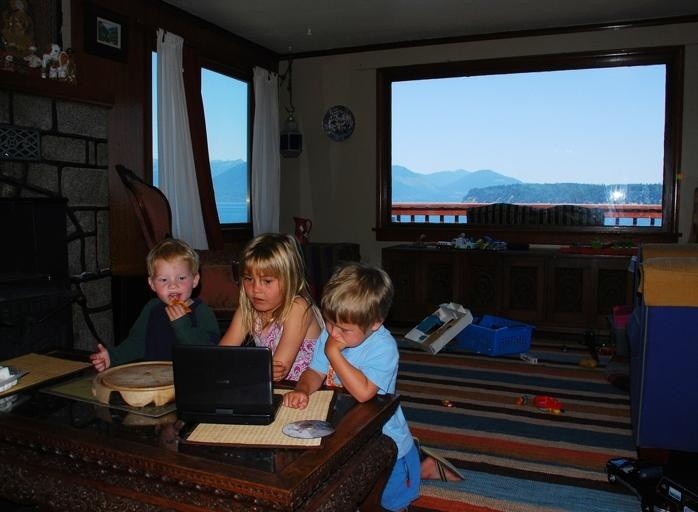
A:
282,61,302,157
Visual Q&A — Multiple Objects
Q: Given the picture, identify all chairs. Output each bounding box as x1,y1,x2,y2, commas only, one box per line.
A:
115,164,240,319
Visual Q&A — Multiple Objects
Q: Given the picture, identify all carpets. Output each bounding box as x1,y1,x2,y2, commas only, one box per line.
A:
395,326,646,512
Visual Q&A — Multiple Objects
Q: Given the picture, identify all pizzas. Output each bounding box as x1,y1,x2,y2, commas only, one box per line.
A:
168,296,193,314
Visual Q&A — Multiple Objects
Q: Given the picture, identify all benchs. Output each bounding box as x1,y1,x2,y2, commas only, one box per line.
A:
467,203,604,226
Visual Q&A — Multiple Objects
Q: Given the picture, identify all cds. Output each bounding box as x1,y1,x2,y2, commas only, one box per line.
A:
282,419,338,440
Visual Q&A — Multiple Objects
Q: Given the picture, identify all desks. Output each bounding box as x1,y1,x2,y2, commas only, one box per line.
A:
0,344,401,512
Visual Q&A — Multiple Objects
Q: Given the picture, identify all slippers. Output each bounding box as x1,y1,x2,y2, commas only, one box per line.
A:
420,445,465,483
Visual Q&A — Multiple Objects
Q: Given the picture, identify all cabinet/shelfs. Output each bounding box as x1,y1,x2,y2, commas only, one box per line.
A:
267,243,361,295
380,240,638,333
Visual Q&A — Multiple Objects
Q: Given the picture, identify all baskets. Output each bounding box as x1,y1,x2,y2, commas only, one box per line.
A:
455,314,537,357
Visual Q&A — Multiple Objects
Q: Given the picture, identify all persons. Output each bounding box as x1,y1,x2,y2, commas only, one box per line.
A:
217,231,326,384
88,238,222,378
281,262,467,512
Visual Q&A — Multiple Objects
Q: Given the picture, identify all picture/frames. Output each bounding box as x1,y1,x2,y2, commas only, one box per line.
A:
84,8,128,64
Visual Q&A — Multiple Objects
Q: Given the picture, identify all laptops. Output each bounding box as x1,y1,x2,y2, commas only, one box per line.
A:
172,343,282,425
